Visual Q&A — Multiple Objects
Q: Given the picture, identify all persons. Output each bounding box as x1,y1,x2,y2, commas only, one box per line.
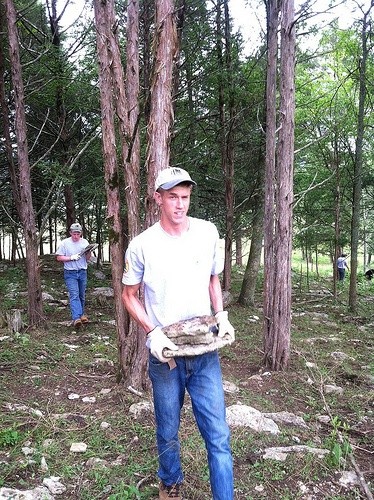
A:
336,254,349,281
120,165,238,500
54,223,92,330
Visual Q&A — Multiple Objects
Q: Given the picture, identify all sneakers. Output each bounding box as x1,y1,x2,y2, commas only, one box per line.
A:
159,481,182,500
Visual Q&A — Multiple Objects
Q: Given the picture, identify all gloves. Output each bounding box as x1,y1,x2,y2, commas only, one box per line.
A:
215,312,236,345
70,253,81,261
146,325,178,362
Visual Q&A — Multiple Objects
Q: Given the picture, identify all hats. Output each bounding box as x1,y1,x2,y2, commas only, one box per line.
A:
70,223,82,233
154,167,197,192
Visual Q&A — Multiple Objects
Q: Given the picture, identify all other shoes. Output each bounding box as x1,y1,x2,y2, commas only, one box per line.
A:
81,316,88,322
74,319,83,326
